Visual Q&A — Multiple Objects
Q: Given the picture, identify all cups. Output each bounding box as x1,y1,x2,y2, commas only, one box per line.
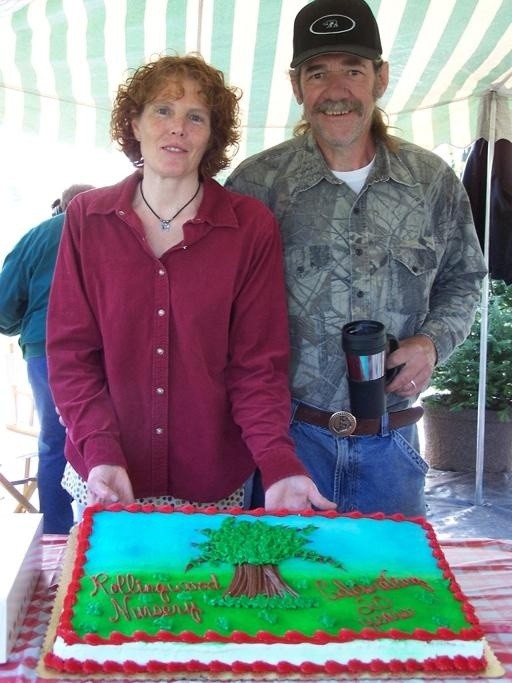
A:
342,318,403,421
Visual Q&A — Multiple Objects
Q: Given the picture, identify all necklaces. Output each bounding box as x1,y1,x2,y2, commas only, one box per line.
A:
140,178,201,231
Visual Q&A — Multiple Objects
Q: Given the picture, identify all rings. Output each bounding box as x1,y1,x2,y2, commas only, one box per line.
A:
410,380,416,389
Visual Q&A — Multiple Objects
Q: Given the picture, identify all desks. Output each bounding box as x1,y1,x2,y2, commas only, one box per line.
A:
1,529,512,681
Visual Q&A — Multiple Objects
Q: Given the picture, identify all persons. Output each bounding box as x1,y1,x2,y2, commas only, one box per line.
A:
223,0,488,520
0,184,96,535
45,46,337,512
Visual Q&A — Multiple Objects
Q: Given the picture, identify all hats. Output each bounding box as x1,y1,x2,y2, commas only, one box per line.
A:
290,1,383,68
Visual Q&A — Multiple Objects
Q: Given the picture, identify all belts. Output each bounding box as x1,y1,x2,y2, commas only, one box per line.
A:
294,402,424,438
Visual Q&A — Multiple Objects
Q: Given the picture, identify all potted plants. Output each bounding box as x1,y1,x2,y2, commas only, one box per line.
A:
421,273,511,471
